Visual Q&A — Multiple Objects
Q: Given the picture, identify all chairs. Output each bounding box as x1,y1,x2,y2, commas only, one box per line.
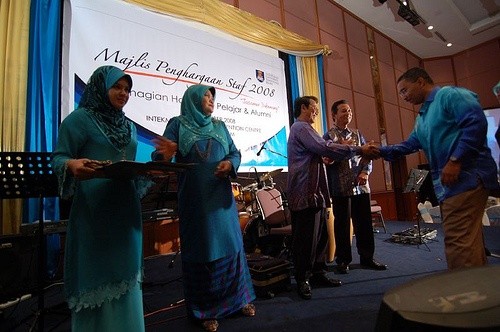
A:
256,186,292,256
370,199,387,234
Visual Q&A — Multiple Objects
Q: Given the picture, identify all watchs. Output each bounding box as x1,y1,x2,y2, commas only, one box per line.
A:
450,157,457,161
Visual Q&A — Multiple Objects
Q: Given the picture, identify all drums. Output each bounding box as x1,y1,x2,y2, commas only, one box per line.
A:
231,182,245,201
140,208,182,260
0,234,37,302
238,211,285,256
242,187,255,202
236,203,246,212
18,219,69,282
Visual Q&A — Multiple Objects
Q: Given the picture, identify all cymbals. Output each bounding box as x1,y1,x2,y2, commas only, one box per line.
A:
238,177,257,179
261,169,283,181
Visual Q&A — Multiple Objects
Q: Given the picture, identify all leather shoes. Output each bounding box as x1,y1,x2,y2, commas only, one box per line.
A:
310,272,343,287
337,261,350,274
298,280,312,298
360,258,388,270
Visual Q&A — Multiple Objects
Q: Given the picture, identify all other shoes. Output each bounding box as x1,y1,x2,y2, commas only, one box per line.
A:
242,304,256,316
203,320,219,332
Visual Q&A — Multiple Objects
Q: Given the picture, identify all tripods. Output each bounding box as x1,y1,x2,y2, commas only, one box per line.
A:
402,168,440,252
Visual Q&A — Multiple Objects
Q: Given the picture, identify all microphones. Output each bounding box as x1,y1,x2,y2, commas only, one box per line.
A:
257,141,267,156
254,168,262,189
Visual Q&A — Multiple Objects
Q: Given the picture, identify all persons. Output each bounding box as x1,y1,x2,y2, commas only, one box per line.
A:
52,65,162,332
323,100,388,274
368,67,497,273
287,96,381,297
151,85,256,332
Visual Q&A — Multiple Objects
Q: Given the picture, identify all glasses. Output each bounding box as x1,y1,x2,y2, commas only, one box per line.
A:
400,81,418,97
